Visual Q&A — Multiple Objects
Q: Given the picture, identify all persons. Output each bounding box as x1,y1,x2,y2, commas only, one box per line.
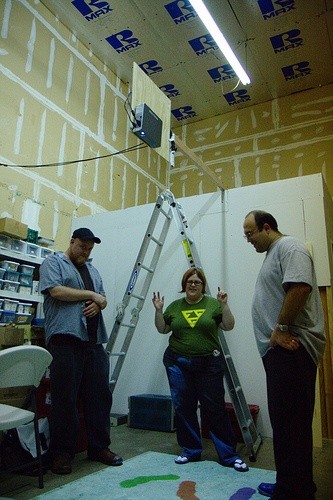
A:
242,210,326,500
151,267,250,471
39,227,124,475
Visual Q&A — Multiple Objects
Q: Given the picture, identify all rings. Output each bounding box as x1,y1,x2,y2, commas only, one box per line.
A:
292,340,294,342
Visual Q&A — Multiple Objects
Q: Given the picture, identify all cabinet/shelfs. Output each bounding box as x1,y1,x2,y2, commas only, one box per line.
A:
0,249,45,304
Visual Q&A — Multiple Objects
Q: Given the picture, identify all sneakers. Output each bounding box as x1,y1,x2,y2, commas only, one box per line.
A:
47,455,72,474
88,448,122,465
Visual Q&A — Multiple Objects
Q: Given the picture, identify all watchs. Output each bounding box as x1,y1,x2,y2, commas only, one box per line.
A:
276,324,288,332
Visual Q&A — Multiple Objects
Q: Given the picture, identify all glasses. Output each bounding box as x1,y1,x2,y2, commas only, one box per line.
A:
243,226,265,239
186,281,203,286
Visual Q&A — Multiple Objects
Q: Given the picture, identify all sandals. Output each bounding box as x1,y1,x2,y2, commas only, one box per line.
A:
175,454,191,463
233,459,248,471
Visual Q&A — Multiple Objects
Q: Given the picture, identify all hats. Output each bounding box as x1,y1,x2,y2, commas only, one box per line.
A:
72,228,101,244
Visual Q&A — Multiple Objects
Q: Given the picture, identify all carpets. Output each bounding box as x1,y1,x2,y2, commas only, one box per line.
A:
33,450,276,500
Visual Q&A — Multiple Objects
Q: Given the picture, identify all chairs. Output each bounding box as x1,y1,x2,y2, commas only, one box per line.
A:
0,346,55,488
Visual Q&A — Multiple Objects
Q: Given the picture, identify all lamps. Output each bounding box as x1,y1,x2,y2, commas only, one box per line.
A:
189,0,251,87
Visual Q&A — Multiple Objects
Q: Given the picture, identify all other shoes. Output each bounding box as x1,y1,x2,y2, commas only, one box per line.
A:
258,482,276,497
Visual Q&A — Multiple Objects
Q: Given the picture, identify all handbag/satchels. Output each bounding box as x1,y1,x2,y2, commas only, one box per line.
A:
16,417,51,458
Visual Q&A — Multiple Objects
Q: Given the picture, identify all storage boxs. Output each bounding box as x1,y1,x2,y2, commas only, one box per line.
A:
0,216,56,325
127,394,179,432
199,402,260,443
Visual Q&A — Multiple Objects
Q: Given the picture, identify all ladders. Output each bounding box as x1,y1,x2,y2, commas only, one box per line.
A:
105,189,264,461
